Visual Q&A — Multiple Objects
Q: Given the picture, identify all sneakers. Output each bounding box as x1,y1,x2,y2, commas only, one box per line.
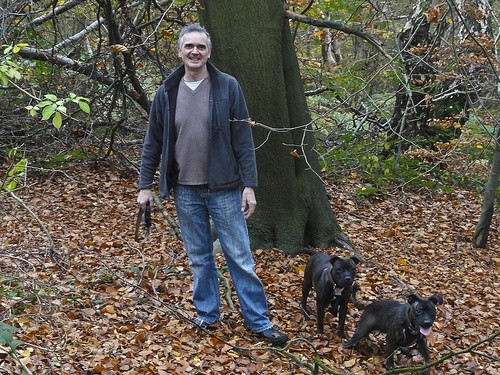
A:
192,318,219,331
257,326,288,345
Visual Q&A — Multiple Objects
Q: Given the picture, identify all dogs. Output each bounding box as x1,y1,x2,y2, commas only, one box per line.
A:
348,294,443,368
302,252,360,342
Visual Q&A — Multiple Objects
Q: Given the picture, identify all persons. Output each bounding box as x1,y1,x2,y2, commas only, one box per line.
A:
136,22,288,346
